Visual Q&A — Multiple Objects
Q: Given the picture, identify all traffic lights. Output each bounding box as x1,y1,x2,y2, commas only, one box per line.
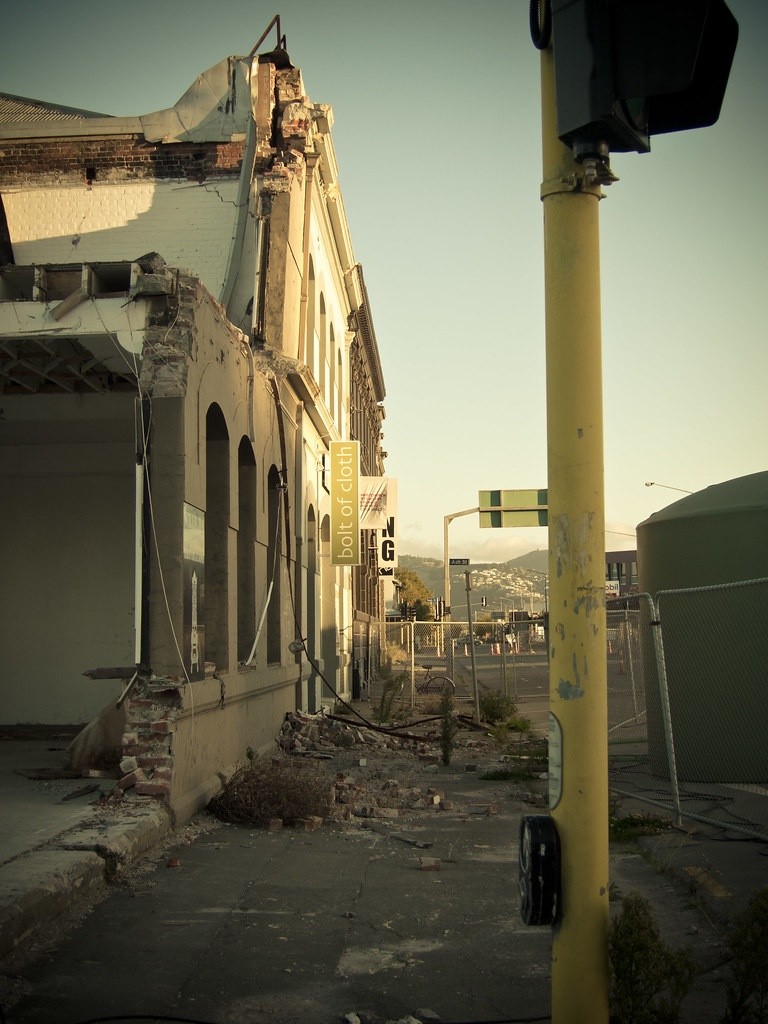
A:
597,0,741,155
